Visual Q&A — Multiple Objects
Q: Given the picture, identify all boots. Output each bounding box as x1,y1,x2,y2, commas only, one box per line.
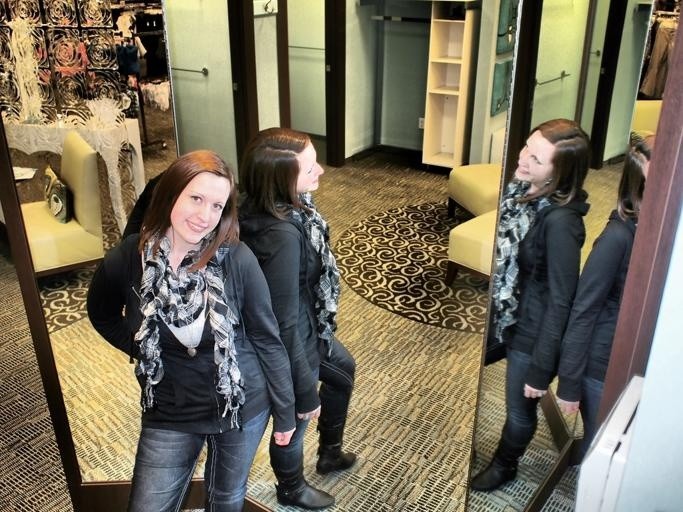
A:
470,440,519,491
274,473,334,508
316,446,356,471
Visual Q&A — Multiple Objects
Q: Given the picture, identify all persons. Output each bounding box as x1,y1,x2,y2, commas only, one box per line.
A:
242,128,358,508
470,119,590,492
557,134,656,454
85,148,298,510
